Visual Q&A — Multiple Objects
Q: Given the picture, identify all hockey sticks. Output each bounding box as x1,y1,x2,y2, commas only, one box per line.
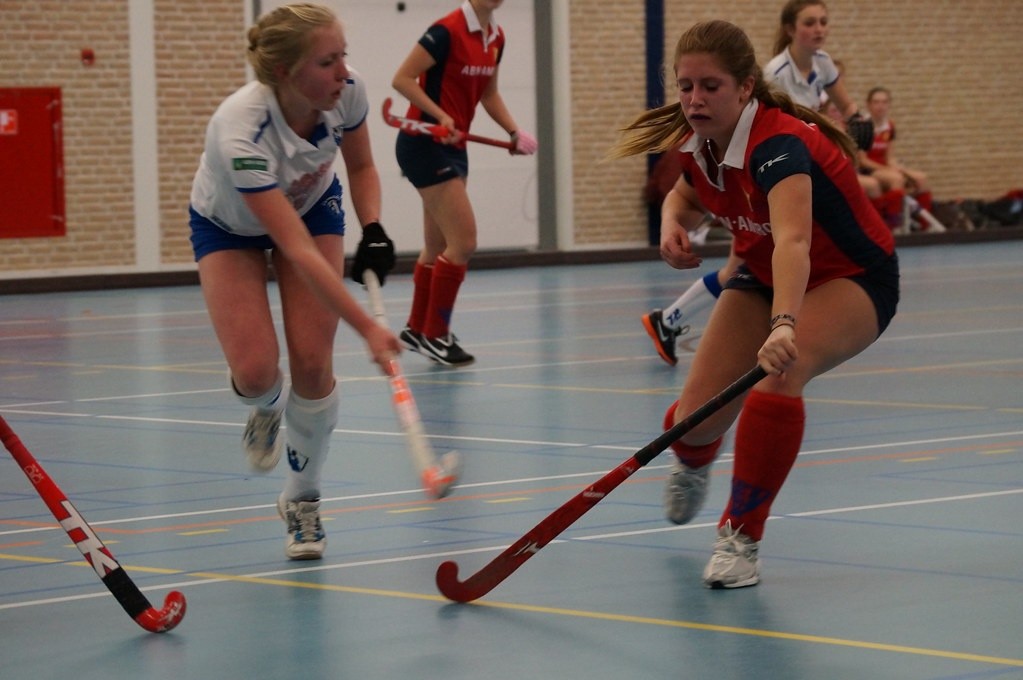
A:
1,405,187,634
381,95,513,150
436,364,770,603
362,269,464,501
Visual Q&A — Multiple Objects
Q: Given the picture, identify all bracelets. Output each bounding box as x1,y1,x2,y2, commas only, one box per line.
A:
768,313,797,335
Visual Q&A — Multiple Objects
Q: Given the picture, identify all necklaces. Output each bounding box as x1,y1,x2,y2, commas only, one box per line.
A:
707,138,718,169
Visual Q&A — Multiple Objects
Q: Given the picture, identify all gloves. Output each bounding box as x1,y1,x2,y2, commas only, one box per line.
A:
844,108,875,152
509,130,538,157
349,223,395,291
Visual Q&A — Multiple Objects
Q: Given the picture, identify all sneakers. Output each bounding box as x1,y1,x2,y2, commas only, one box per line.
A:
397,323,443,365
277,488,328,560
419,332,476,367
701,518,760,590
660,453,714,526
240,400,286,473
642,308,691,367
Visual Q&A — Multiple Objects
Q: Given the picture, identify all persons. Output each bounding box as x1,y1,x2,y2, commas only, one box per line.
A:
612,0,932,587
186,1,537,559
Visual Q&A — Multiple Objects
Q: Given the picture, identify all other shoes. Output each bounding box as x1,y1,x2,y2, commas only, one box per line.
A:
917,223,946,234
890,225,910,236
